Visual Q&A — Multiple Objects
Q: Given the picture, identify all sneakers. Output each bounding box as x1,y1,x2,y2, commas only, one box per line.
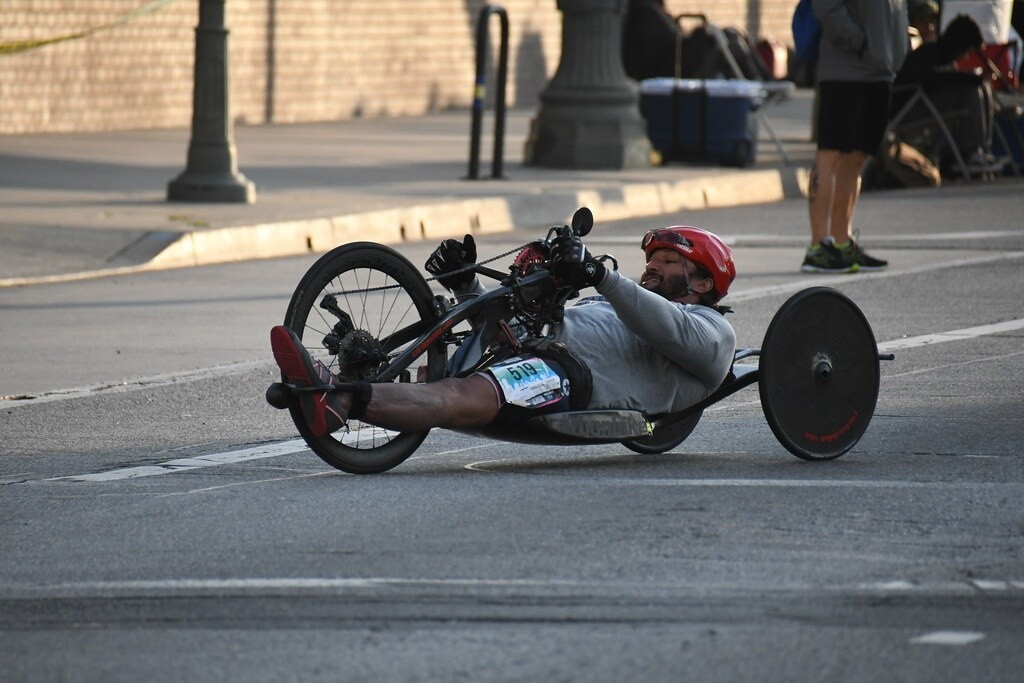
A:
270,326,357,436
836,229,888,273
801,239,859,274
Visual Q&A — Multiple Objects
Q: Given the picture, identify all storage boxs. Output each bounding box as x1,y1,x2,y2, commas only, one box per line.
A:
643,79,769,171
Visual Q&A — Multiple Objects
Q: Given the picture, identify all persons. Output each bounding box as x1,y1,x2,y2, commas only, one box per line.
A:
800,1,909,273
890,10,1000,166
271,225,736,434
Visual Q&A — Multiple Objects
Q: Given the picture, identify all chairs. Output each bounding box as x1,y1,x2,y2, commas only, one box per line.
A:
879,24,1024,182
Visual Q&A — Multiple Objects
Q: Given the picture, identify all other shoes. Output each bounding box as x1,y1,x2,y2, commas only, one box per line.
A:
950,146,1010,174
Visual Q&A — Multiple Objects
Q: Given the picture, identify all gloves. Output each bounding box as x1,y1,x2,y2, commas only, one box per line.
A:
424,234,477,293
551,225,606,292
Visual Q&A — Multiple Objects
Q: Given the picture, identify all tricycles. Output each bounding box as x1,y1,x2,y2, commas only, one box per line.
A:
263,206,896,475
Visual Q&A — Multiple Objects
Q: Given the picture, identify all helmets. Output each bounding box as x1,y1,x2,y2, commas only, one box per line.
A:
646,225,736,298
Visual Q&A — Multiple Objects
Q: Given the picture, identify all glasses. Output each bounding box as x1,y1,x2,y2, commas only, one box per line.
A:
641,229,692,250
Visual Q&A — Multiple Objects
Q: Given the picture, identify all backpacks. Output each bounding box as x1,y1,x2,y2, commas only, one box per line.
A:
790,0,823,70
